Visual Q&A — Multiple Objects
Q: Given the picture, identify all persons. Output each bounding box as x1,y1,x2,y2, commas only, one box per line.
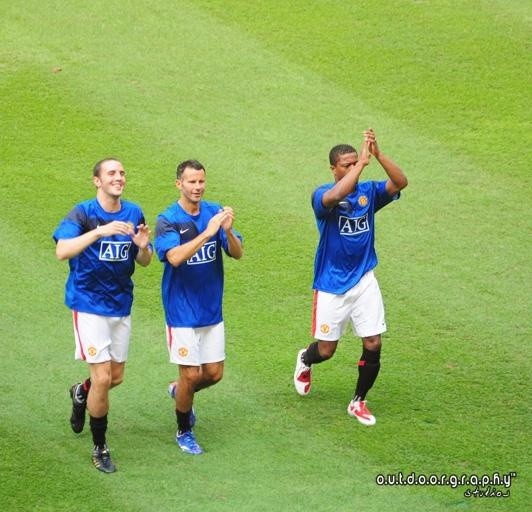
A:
294,129,410,423
150,159,246,457
55,159,154,474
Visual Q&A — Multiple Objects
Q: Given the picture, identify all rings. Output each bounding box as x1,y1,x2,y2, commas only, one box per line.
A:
127,232,130,235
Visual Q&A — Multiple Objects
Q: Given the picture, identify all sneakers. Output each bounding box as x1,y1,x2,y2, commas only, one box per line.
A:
175,428,203,457
167,381,196,427
90,443,117,475
345,399,377,428
68,383,88,433
293,348,313,397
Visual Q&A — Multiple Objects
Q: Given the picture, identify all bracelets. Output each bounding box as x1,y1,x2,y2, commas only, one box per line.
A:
98,224,105,237
